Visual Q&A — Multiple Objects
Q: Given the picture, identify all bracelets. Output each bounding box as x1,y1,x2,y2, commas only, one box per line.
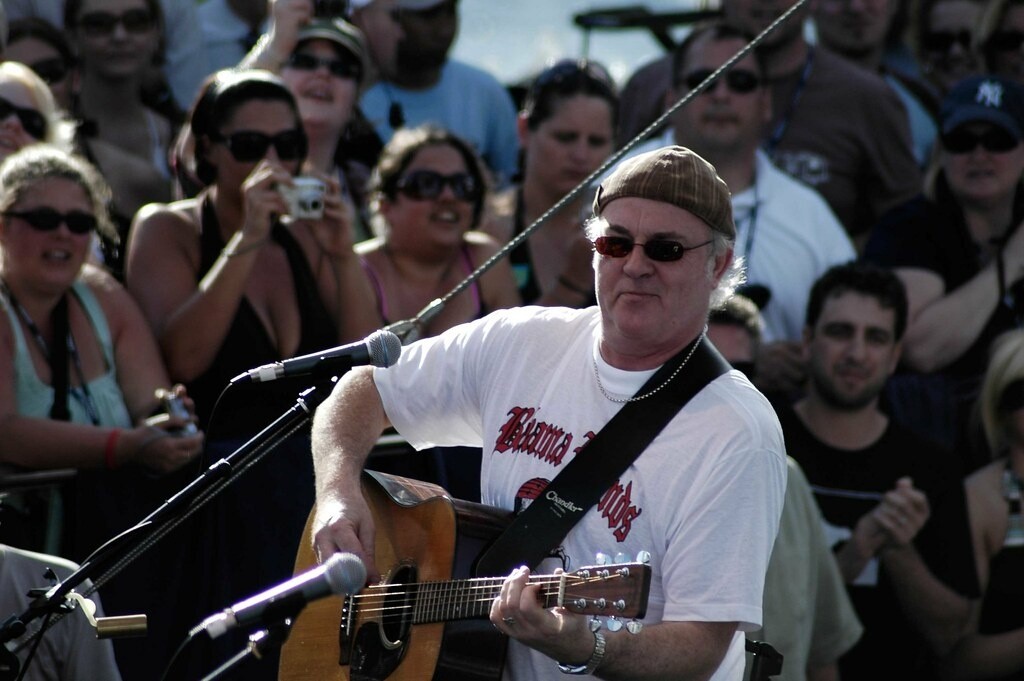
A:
107,426,121,470
557,627,608,677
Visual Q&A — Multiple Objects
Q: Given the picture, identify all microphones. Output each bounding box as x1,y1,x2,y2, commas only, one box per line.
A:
230,329,402,385
189,552,367,643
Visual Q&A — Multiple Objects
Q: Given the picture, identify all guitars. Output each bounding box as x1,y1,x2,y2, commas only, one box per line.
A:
278,468,653,681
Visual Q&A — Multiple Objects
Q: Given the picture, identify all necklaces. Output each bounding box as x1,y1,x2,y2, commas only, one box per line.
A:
379,234,458,286
592,323,708,405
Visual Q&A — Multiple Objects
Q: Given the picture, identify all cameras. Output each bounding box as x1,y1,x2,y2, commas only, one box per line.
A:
149,392,195,435
275,175,326,221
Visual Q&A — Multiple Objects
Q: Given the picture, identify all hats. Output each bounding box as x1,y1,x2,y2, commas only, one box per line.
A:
940,76,1024,138
396,0,441,9
297,16,366,61
592,146,736,241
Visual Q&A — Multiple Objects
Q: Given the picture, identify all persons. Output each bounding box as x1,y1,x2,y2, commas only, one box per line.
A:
0,0,1023,681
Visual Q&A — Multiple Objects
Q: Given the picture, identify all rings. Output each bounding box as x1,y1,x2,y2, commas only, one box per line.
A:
898,517,905,526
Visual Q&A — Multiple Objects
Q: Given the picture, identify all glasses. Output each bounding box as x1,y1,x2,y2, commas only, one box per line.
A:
0,98,45,141
76,9,153,34
953,129,1018,152
592,237,715,262
393,170,478,202
554,59,612,87
217,131,308,162
3,208,97,235
293,55,358,78
678,69,757,94
